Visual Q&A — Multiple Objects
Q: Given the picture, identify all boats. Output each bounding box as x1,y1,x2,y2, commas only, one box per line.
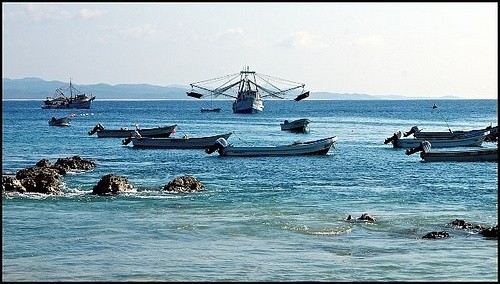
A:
39,79,98,109
199,107,223,113
405,141,498,162
87,124,179,139
47,117,72,126
204,133,338,158
280,118,311,133
383,131,489,149
403,126,500,139
122,131,235,149
186,66,311,114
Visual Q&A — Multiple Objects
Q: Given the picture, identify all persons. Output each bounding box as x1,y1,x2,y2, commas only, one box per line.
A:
134,131,142,139
